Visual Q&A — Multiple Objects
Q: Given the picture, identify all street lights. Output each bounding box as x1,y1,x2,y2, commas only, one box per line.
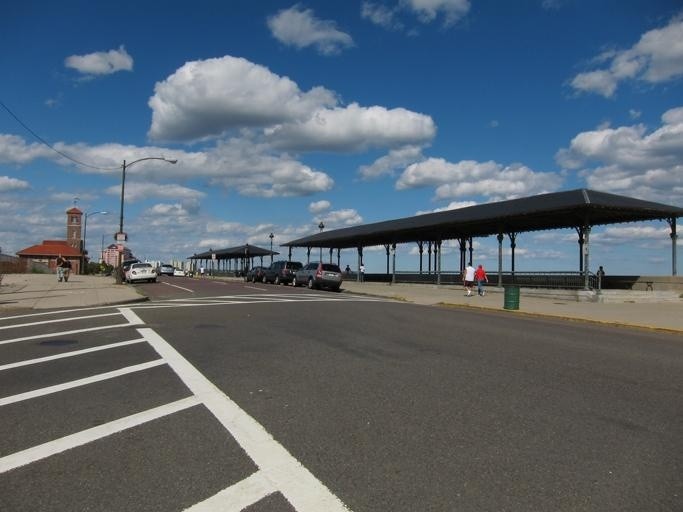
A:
319,222,324,263
270,233,274,264
81,211,108,275
116,158,177,284
391,246,396,281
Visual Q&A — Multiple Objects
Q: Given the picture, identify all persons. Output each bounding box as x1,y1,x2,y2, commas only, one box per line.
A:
200,265,205,279
597,266,605,275
62,257,72,282
55,252,66,282
345,265,352,271
475,264,488,296
462,262,475,297
360,264,365,275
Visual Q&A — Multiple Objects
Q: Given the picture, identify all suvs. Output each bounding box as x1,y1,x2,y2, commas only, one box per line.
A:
263,261,302,285
292,262,343,289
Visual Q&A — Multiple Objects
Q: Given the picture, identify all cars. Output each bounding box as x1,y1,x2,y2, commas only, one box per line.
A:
121,261,187,284
247,266,267,283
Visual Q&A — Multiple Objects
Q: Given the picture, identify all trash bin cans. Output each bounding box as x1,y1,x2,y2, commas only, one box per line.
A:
502,284,521,310
